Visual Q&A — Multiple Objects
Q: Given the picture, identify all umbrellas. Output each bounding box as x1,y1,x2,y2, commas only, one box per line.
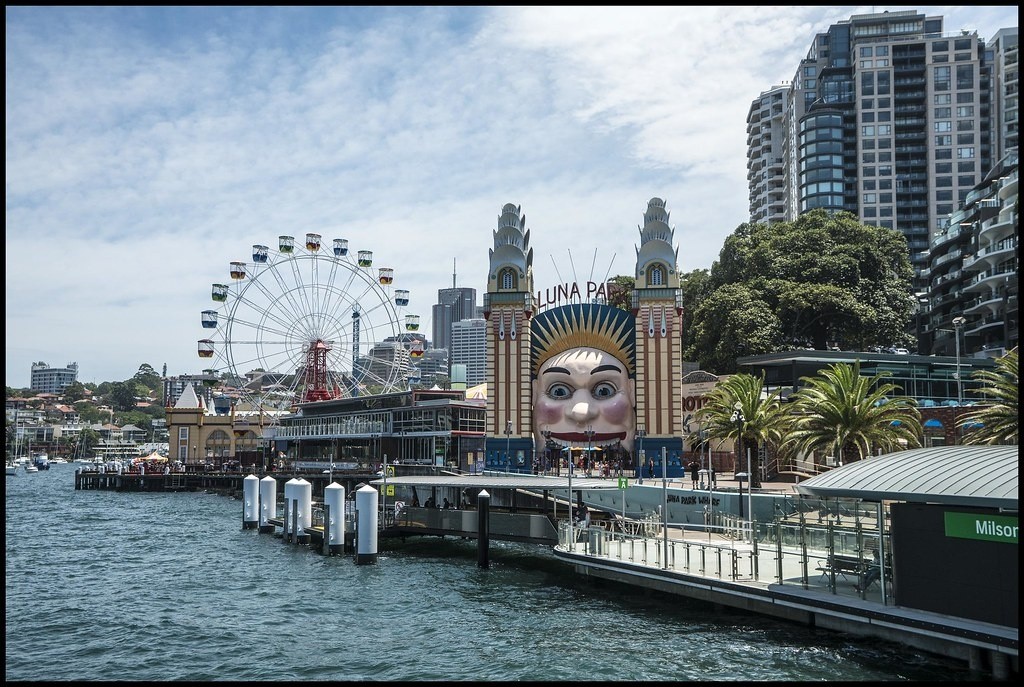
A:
140,452,168,461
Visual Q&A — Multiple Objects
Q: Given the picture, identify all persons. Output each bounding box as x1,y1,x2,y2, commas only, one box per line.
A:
534,455,625,478
222,460,238,471
853,549,886,593
576,502,590,529
688,460,700,490
130,461,175,472
649,457,655,480
410,495,467,509
706,462,717,491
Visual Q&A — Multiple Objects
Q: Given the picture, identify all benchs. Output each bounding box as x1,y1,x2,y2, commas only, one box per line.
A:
815,554,895,599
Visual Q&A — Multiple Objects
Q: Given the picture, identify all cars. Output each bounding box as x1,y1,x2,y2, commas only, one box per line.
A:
891,349,909,355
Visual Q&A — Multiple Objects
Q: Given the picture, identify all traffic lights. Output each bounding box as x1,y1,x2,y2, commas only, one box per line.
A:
503,420,514,467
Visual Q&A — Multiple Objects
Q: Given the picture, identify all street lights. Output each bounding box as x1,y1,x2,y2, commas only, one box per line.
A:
131,439,146,455
698,429,709,491
585,431,596,476
193,445,196,459
636,429,647,485
219,446,226,473
729,414,746,517
540,430,551,475
236,444,244,472
261,441,268,472
204,446,211,465
328,436,340,470
370,434,379,466
952,316,967,403
293,439,300,475
398,432,407,464
